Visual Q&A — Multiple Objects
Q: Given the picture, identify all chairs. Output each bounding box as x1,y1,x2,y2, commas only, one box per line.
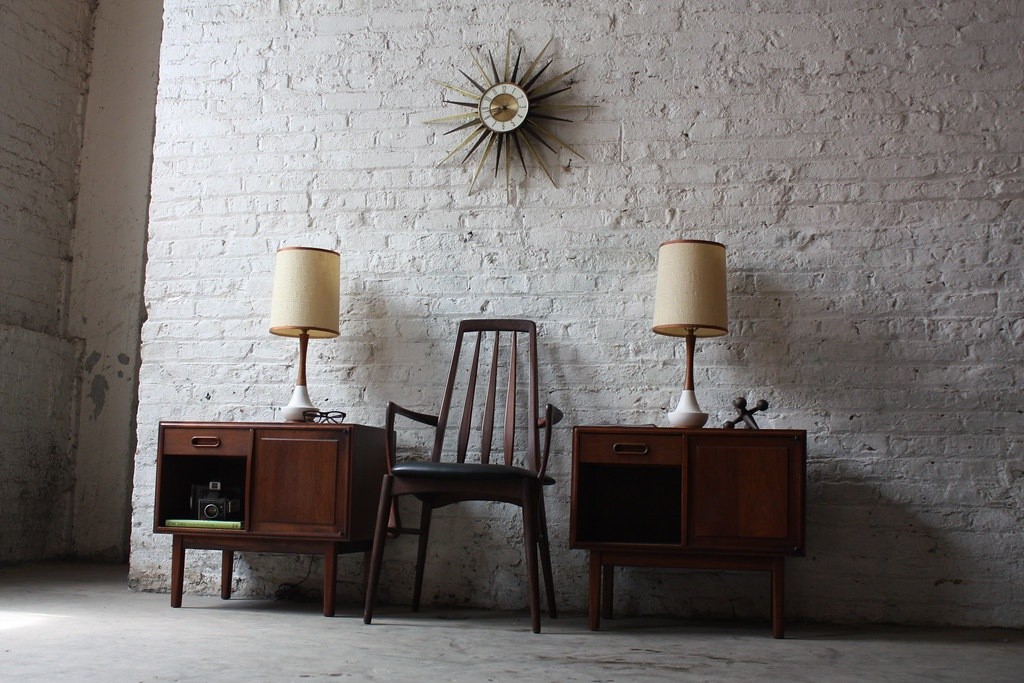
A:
363,319,564,633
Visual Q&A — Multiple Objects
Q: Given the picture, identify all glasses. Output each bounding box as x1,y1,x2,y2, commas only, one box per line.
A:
302,410,346,425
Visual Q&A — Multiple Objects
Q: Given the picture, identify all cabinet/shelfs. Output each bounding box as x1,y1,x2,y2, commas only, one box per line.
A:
154,421,397,617
568,426,806,638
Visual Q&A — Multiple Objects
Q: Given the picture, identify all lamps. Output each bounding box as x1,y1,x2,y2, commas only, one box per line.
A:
269,247,342,421
651,240,729,427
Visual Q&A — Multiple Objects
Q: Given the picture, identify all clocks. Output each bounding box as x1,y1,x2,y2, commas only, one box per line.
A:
478,83,529,133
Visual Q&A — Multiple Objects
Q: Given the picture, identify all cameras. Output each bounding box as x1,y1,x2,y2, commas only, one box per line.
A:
190,482,241,522
